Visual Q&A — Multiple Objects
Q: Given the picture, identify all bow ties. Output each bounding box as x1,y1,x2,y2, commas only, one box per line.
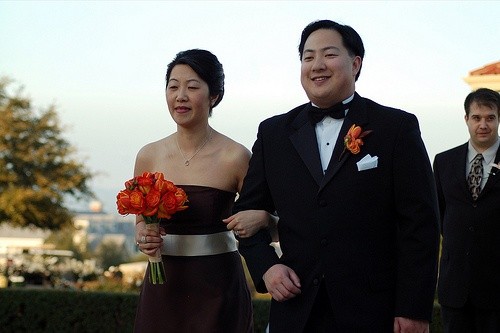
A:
307,101,352,127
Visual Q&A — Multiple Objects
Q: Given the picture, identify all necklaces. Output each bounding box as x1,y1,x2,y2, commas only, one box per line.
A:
175,127,213,167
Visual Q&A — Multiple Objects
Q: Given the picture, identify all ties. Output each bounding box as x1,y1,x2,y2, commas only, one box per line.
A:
465,153,484,204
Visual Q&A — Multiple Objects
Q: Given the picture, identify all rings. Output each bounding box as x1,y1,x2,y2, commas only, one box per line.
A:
235,231,240,237
141,237,146,244
136,242,141,248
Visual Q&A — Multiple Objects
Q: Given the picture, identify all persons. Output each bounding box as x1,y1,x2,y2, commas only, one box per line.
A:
232,20,441,333
433,89,500,333
3,254,102,290
134,49,279,333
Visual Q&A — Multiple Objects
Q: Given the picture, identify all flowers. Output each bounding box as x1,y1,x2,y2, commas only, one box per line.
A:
339,124,364,160
117,172,189,285
